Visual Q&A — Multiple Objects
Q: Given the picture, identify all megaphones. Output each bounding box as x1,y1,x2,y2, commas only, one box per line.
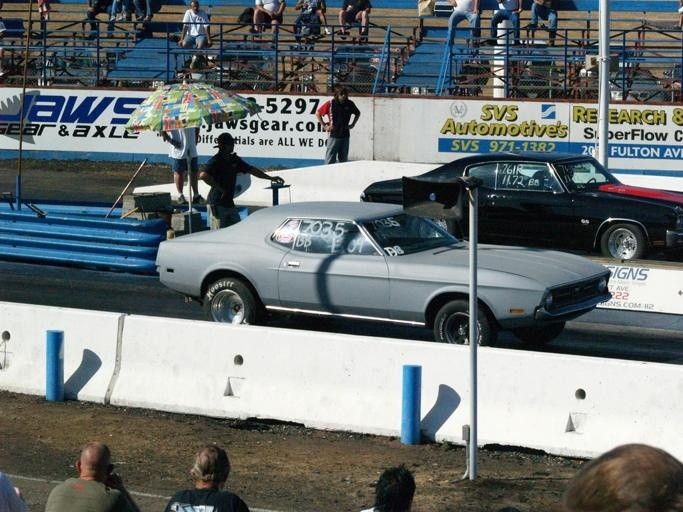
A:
403,176,482,220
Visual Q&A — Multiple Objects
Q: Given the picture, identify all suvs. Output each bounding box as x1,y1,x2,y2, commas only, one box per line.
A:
320,45,383,93
206,43,274,89
35,45,105,88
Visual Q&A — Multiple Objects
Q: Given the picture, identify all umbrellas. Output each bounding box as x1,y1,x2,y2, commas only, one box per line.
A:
124,80,261,234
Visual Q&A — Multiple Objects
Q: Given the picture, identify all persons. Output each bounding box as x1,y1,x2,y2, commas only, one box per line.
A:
445,0,557,47
0,470,31,511
358,462,416,512
177,2,212,69
252,1,373,50
201,131,280,231
45,441,140,511
314,87,360,165
562,444,683,512
87,1,162,37
164,443,250,512
160,127,203,203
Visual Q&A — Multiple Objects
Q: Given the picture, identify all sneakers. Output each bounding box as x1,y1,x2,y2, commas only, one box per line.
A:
192,194,207,204
177,194,188,204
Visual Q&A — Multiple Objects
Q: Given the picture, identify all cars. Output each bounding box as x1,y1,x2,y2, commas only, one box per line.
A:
658,62,681,101
511,40,559,98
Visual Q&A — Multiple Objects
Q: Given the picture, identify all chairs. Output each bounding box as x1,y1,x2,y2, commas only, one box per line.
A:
530,171,543,189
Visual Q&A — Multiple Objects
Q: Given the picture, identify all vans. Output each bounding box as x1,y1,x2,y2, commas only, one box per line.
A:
610,67,665,103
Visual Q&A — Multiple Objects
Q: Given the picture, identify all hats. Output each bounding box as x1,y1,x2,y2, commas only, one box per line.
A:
213,132,240,148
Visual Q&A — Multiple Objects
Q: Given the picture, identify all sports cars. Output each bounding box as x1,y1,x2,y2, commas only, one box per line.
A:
357,148,683,262
155,199,611,345
